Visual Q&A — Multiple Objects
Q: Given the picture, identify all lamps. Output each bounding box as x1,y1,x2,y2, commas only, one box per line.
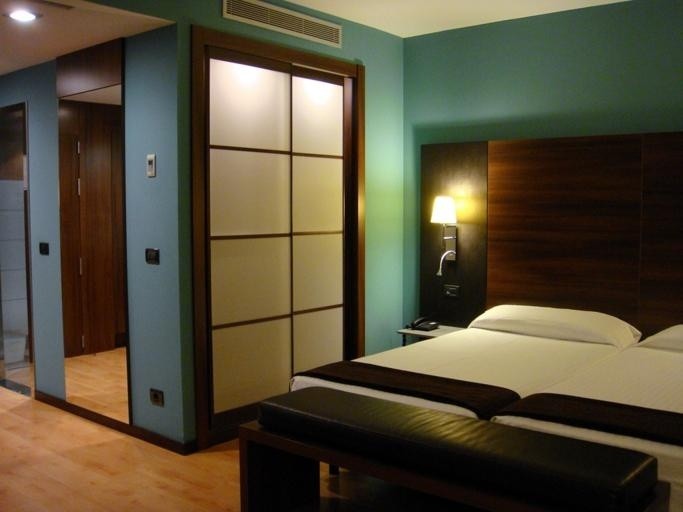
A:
428,193,460,278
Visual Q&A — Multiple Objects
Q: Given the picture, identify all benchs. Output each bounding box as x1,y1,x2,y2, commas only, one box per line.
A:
237,384,672,511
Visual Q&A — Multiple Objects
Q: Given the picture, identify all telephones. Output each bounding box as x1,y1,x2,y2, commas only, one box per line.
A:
411,316,440,331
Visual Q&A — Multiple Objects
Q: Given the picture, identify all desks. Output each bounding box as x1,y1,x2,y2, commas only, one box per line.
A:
394,324,464,347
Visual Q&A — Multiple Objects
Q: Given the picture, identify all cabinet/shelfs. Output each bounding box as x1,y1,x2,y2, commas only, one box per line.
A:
57,98,125,361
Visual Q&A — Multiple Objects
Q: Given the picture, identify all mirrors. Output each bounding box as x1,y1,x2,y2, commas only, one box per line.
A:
0,98,35,399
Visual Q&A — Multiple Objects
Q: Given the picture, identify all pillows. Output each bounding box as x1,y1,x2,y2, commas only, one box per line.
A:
632,320,682,351
464,302,645,348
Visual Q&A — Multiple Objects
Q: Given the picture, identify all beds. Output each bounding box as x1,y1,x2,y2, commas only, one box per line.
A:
287,132,683,510
